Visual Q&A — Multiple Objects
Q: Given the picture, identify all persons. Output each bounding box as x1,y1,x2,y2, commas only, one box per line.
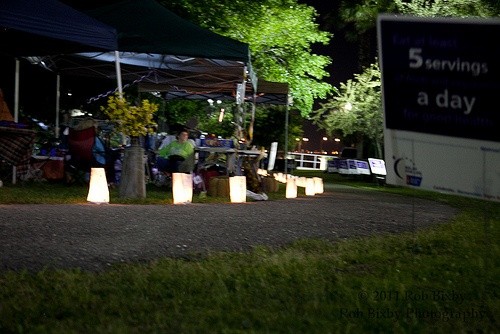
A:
157,128,179,151
155,129,196,192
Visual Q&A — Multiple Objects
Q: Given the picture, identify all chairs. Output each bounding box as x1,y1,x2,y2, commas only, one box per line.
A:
33,135,208,186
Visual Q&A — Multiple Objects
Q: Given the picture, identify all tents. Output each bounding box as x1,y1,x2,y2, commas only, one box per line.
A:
136,74,290,182
51,0,259,180
0,0,127,148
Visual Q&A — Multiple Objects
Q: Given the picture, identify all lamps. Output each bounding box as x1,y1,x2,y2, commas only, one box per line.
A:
171,173,193,206
228,176,246,204
257,168,323,198
86,168,111,204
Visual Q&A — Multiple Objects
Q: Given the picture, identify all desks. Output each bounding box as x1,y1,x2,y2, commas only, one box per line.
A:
197,147,269,200
